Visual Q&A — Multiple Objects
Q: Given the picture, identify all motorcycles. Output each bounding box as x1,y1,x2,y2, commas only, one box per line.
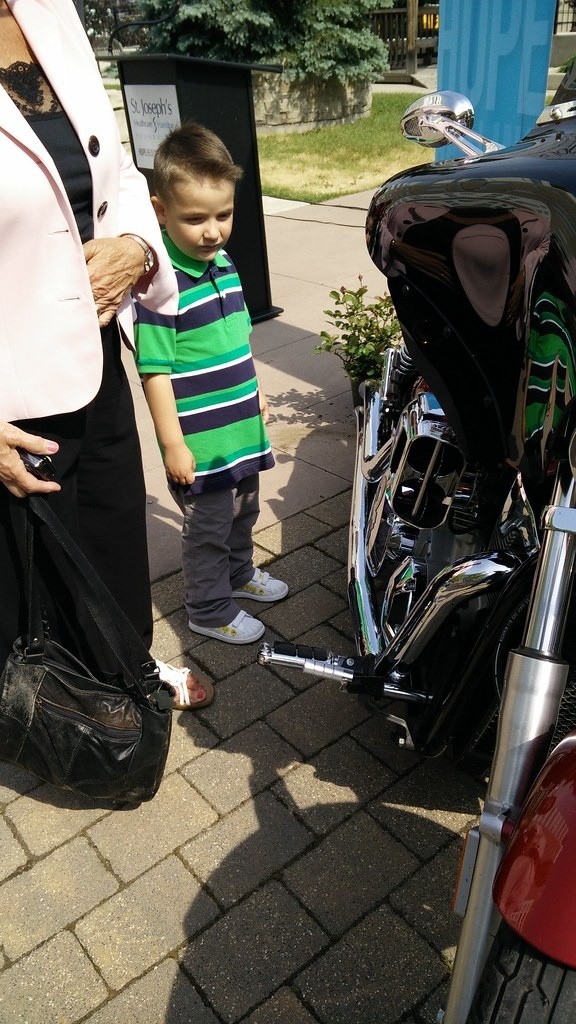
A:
258,57,576,1024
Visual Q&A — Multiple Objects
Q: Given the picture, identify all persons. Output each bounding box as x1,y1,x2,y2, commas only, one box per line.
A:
128,124,289,643
0,0,215,711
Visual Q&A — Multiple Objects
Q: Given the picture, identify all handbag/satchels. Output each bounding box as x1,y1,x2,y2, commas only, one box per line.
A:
0,449,176,804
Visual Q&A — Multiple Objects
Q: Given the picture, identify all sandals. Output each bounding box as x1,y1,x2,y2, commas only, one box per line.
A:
153,658,215,709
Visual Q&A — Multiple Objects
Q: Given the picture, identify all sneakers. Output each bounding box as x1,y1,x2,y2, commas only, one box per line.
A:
189,609,265,644
231,568,288,602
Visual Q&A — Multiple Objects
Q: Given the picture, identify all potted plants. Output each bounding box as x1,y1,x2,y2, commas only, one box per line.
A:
310,272,405,409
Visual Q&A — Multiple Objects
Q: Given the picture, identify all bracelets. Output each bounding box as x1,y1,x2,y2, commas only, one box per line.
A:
121,234,155,276
